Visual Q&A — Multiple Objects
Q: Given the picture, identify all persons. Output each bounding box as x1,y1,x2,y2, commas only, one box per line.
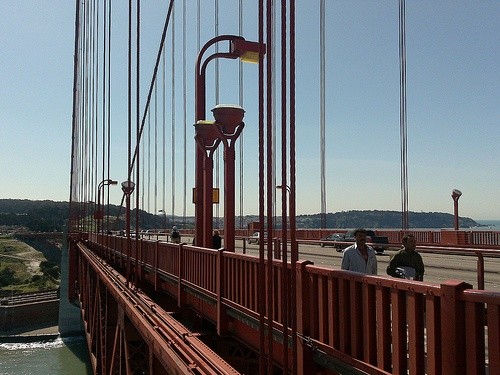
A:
170,225,222,250
386,233,425,281
341,229,377,275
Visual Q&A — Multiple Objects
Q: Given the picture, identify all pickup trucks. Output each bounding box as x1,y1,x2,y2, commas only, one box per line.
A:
334,229,388,253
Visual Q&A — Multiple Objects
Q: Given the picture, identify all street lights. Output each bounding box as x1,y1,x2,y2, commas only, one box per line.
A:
451,187,462,230
94,178,136,236
187,33,266,253
118,180,135,236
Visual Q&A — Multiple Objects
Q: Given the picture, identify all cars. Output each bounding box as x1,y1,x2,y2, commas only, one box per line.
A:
248,231,267,243
319,233,343,246
108,229,135,236
